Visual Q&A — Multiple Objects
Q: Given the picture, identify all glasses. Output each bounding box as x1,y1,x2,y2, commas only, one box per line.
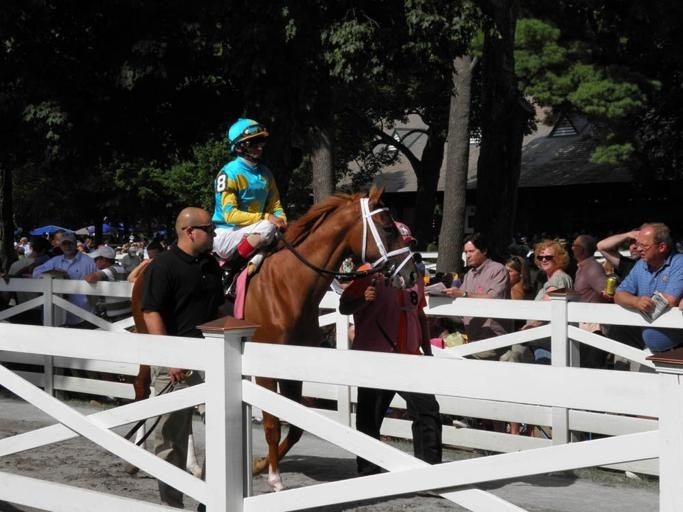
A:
182,226,212,233
537,256,553,260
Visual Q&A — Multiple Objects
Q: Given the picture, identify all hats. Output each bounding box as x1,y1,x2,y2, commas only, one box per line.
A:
60,233,76,244
394,221,420,245
86,246,116,259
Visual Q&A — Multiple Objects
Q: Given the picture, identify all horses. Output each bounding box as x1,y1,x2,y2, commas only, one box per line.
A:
129,185,419,494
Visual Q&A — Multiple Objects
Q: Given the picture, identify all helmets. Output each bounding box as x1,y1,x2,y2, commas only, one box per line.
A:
228,118,269,152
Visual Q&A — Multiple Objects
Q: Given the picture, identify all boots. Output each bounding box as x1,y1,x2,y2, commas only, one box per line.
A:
221,246,247,300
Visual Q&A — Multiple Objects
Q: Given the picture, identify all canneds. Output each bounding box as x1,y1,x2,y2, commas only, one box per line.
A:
606,277,617,295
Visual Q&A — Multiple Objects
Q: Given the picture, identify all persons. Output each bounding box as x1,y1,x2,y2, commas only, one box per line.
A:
337,221,442,475
139,206,225,512
0,221,682,481
210,118,287,288
